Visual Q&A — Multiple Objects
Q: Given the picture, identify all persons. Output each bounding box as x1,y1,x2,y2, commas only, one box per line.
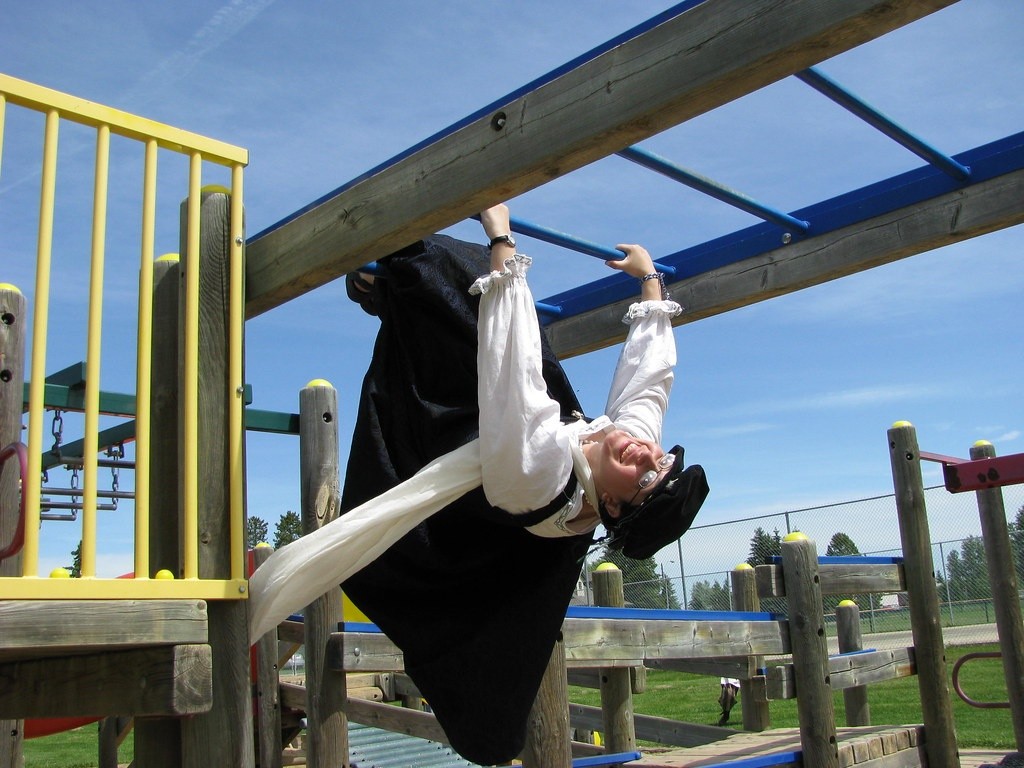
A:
247,203,710,766
719,677,740,724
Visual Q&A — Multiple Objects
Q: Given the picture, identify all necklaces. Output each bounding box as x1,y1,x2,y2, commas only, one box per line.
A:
583,440,599,507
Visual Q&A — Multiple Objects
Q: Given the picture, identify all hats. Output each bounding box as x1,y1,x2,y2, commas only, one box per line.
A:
622,445,710,560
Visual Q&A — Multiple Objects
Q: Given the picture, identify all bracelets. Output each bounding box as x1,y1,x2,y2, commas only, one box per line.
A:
640,273,672,301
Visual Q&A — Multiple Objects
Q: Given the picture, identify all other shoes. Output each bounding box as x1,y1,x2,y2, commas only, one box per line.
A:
345,272,377,317
719,710,729,725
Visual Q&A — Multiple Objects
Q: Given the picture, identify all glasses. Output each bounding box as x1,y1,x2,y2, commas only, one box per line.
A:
626,452,676,507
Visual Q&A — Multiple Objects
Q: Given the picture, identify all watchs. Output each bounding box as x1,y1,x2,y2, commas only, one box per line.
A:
488,235,515,250
